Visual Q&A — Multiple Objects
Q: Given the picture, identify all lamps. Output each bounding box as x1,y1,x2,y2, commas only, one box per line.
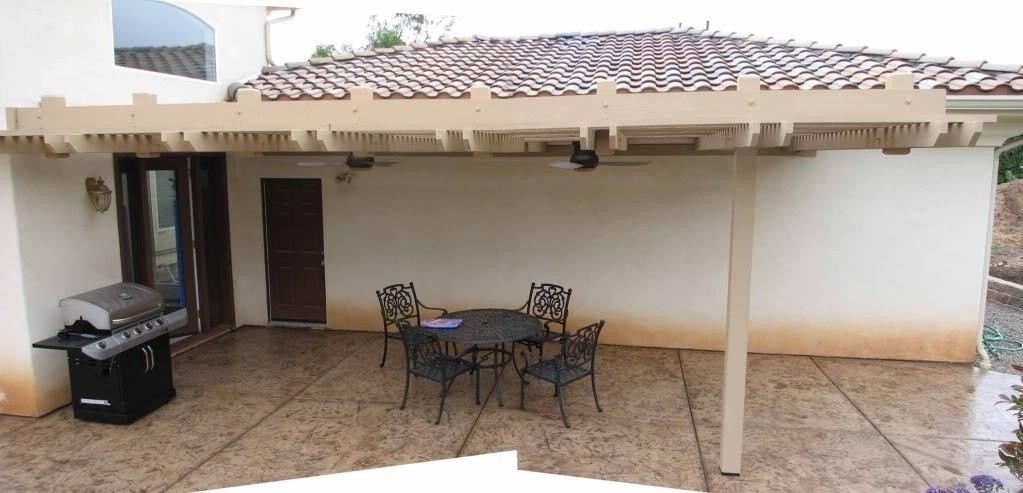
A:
84,178,112,213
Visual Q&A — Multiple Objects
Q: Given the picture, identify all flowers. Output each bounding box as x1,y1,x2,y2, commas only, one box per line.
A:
544,150,650,171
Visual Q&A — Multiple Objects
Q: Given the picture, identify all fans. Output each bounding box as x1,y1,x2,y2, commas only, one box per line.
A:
298,153,403,178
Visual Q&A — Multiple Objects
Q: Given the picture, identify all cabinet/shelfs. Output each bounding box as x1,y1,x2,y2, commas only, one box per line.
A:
67,331,177,425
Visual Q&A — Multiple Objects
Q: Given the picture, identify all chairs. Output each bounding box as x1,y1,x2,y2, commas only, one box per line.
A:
375,281,605,429
